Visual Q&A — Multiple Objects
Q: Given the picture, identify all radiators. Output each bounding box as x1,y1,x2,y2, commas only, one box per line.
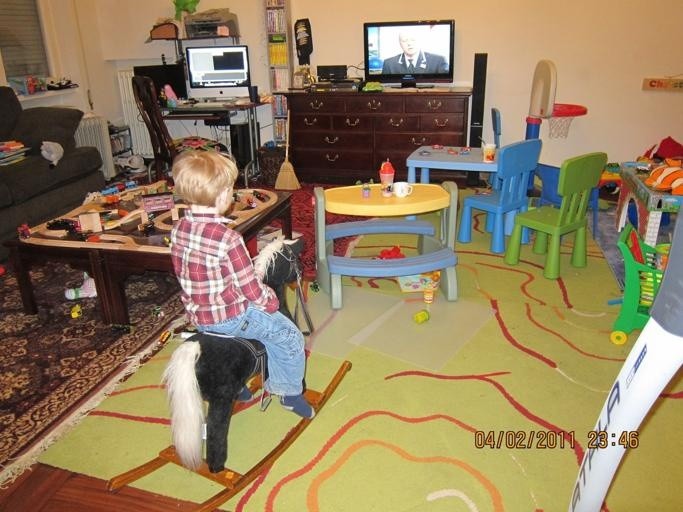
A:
71,113,116,185
114,67,154,157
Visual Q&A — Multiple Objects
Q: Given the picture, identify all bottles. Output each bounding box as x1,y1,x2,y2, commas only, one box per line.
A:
378,158,394,196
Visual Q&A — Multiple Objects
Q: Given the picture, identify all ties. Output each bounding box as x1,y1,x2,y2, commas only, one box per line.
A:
408,59,414,73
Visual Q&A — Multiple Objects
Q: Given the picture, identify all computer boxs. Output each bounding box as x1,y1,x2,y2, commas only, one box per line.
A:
230,121,261,171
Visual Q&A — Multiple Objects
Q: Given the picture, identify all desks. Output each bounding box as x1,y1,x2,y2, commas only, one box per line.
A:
105,124,135,159
402,142,502,223
614,158,683,268
305,175,466,310
134,93,271,190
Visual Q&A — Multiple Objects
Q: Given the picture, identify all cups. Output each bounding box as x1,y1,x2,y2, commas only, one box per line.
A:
248,85,258,103
393,182,412,198
481,144,496,162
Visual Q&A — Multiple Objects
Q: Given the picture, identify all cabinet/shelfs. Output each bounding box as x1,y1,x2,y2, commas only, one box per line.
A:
268,86,476,185
263,0,293,145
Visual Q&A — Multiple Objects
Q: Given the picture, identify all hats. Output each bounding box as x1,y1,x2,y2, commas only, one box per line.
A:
117,154,147,173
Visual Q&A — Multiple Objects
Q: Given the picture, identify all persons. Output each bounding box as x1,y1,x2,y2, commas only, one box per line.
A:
382,32,449,75
169,148,316,420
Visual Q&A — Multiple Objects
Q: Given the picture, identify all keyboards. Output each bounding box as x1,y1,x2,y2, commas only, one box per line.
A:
192,103,231,107
166,112,215,118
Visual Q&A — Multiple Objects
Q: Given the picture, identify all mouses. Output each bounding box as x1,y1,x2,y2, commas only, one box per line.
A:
235,99,246,105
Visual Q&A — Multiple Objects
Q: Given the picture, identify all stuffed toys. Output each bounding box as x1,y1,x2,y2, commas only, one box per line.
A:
65,273,98,301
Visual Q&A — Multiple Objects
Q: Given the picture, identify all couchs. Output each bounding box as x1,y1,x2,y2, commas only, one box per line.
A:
0,84,105,268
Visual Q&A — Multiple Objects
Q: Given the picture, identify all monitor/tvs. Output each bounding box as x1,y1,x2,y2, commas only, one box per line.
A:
133,64,188,101
362,19,455,89
185,45,251,101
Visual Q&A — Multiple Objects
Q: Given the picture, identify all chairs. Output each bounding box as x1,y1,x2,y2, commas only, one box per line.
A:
530,160,605,245
490,107,507,192
129,74,229,187
500,150,611,281
610,221,670,346
455,136,544,257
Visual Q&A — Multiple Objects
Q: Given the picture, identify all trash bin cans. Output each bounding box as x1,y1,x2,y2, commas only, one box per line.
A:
257,147,285,188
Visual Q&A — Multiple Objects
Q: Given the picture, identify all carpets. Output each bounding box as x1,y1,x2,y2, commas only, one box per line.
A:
235,182,373,283
0,225,304,498
583,204,676,297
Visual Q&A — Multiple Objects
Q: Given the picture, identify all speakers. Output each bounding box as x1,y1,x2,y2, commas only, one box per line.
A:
248,86,258,102
468,52,489,190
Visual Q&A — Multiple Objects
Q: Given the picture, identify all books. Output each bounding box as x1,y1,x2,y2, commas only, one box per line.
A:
263,0,289,147
0,140,31,166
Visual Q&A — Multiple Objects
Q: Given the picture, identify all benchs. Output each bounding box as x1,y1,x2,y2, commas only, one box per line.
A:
322,218,438,243
319,247,458,310
